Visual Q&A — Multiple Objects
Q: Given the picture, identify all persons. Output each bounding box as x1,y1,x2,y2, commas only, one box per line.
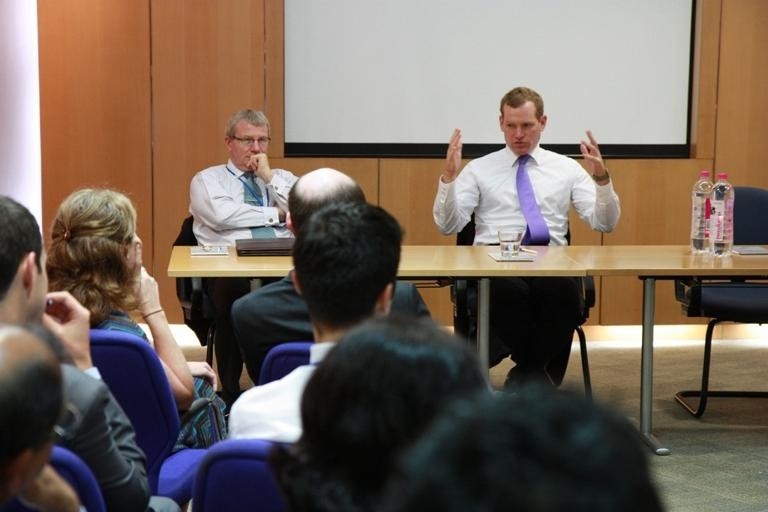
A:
229,201,406,443
271,313,485,511
0,194,181,511
387,381,665,511
186,108,305,405
230,165,432,386
431,86,621,389
0,323,89,512
45,184,230,450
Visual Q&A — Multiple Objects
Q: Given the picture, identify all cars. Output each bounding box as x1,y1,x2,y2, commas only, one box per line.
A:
499,227,524,257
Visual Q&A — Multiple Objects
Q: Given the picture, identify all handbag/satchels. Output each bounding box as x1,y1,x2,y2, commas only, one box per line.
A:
143,308,163,319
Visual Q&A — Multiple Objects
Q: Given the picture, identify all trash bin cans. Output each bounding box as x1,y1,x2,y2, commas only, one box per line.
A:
592,167,610,182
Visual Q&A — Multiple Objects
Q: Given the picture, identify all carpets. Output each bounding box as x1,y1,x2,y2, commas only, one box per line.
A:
244,172,277,240
516,154,549,245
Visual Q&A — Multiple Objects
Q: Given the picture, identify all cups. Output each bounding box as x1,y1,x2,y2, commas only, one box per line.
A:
232,135,271,145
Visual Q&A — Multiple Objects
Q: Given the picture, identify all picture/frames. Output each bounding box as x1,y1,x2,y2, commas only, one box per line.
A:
168,245,587,388
563,244,768,456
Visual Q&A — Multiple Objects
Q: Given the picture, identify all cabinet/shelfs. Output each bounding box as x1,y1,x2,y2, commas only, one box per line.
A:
690,171,714,252
710,173,735,258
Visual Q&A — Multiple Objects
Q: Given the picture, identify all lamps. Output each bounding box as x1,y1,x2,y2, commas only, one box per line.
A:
90,328,224,505
260,343,309,385
172,215,216,367
49,446,103,512
191,438,291,512
455,212,595,405
674,186,768,417
